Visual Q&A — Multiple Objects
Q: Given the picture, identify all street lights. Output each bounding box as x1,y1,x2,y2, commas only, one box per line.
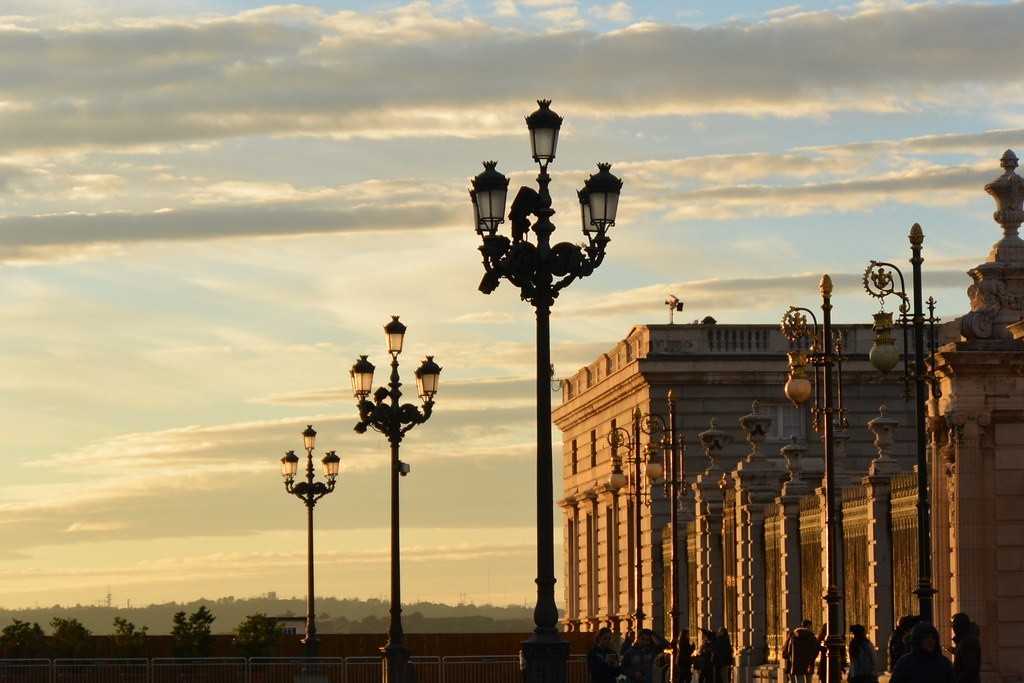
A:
605,404,655,645
862,222,942,624
779,274,850,683
349,315,444,683
280,425,341,683
639,389,690,683
466,98,625,683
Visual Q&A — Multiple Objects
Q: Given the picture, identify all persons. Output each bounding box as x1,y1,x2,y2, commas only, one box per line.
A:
781,619,847,683
675,629,696,683
887,613,928,671
588,627,622,683
620,629,671,683
888,622,953,683
944,612,982,683
696,625,733,683
847,624,878,683
